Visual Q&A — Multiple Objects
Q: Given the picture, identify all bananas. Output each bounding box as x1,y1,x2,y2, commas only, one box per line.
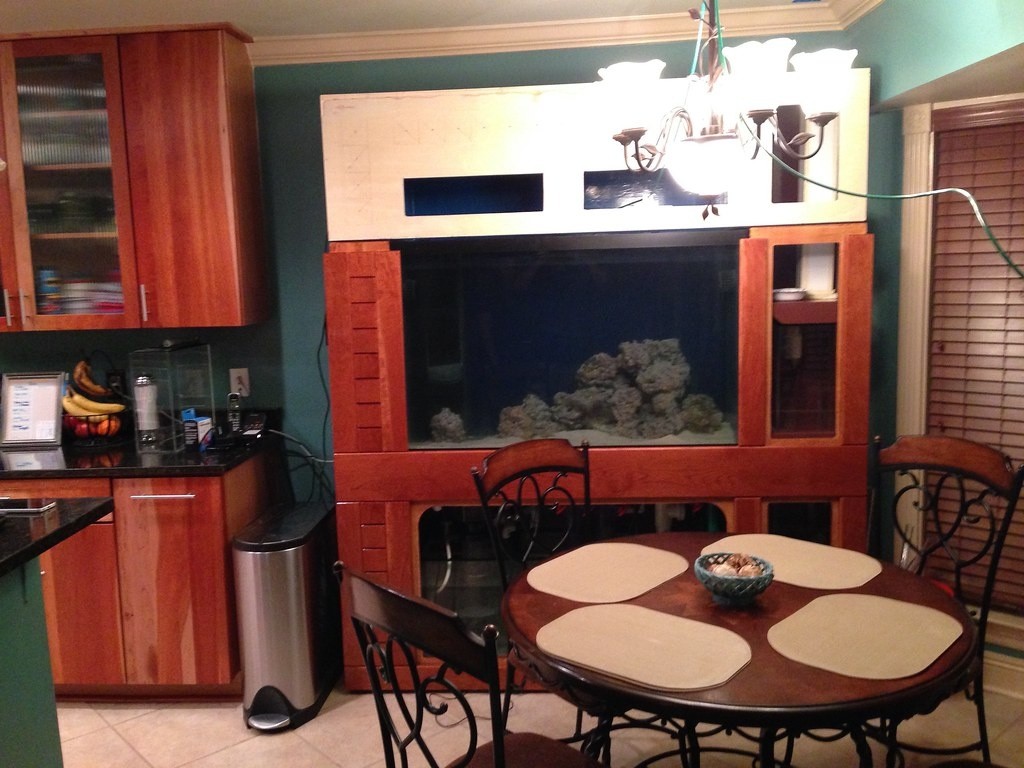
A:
62,386,125,422
74,362,113,396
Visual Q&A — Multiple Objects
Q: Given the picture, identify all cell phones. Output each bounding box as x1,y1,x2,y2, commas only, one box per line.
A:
0,498,56,514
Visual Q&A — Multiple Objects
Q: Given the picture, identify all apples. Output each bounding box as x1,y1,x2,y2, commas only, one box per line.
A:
75,422,96,436
64,413,79,428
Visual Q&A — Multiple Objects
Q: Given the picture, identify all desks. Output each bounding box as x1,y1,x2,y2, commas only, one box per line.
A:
864,430,1024,768
503,531,981,768
333,559,601,768
470,436,593,768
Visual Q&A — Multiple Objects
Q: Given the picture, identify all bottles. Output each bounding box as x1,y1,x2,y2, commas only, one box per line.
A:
134,372,158,445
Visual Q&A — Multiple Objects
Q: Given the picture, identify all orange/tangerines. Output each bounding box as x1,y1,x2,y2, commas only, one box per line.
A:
97,418,120,434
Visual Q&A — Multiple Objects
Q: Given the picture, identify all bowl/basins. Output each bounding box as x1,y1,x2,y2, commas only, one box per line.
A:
774,288,807,301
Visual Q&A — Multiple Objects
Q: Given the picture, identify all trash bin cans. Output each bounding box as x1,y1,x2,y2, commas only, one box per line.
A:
232,498,337,733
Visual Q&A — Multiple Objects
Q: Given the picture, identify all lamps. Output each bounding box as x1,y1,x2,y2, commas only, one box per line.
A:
590,0,859,175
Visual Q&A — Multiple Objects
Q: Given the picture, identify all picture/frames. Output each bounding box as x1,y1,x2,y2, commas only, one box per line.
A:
0,447,66,469
0,371,65,449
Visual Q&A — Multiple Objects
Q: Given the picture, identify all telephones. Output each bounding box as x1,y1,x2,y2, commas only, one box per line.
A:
227,392,267,439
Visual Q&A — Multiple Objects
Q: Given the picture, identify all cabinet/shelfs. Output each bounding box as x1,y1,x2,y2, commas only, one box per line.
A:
0,478,126,704
117,21,270,331
0,23,140,334
0,553,66,768
112,451,267,702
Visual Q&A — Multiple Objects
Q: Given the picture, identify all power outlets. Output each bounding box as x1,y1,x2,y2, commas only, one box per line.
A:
229,368,250,397
